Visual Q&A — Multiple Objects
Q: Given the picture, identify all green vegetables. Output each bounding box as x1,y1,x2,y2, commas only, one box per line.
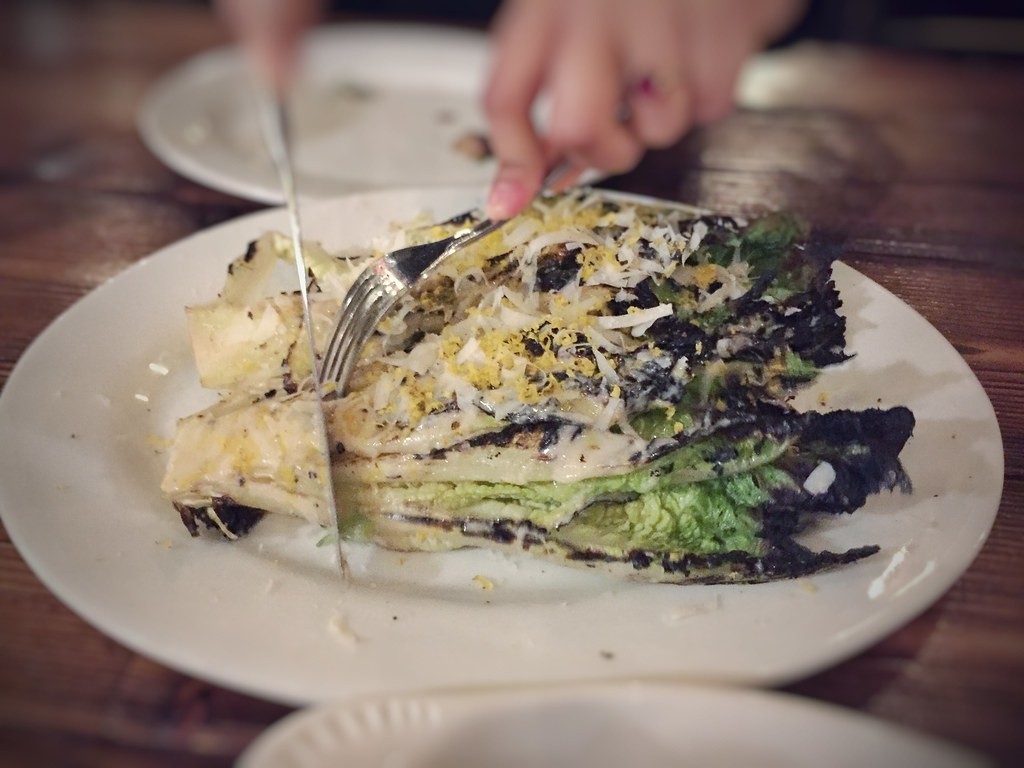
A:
164,193,918,584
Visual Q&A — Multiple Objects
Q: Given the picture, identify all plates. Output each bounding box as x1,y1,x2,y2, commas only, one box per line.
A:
137,25,615,204
0,190,1005,705
231,680,1000,768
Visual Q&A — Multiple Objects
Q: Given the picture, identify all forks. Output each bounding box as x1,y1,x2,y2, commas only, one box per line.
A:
320,156,581,391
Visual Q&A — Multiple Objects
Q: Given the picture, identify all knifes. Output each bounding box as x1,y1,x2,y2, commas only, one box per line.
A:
261,87,344,568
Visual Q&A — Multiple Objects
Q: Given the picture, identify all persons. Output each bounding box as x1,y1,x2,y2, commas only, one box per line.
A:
218,0,811,221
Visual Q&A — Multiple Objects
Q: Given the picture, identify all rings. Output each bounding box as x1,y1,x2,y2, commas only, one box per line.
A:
625,68,678,106
